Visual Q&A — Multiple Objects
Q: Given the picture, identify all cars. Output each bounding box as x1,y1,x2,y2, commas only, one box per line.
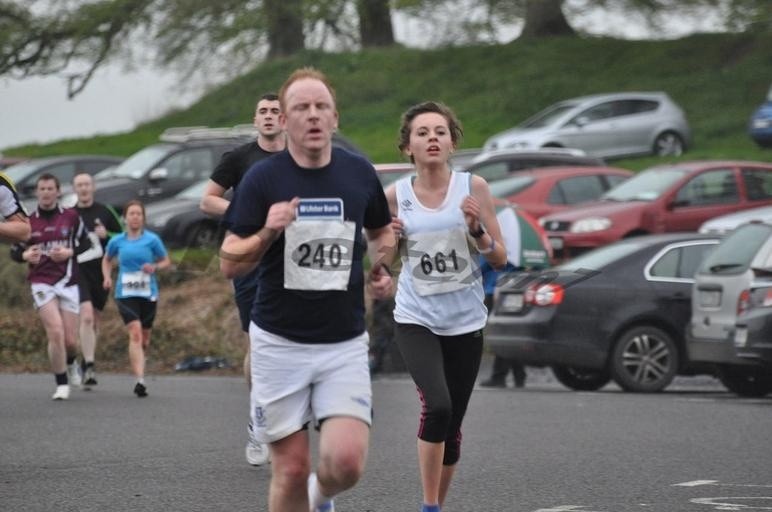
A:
369,162,416,189
141,178,235,246
731,267,772,361
485,167,635,222
0,158,22,171
484,228,726,398
695,202,772,236
3,153,123,213
479,89,696,162
745,92,771,148
446,146,595,181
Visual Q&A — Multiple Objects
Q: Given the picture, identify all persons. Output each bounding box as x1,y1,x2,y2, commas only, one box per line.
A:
101,201,170,398
379,101,508,512
477,250,527,388
1,172,33,242
201,93,290,467
8,173,84,400
66,170,124,391
218,71,397,512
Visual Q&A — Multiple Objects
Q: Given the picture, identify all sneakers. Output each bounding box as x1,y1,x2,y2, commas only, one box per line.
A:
246,423,269,465
133,381,148,396
514,369,525,387
308,473,335,512
54,359,97,400
480,375,505,387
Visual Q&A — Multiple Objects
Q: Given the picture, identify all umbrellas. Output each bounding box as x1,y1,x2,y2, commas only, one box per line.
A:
485,196,554,267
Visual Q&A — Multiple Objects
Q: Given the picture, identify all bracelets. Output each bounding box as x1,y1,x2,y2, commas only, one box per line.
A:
152,262,159,272
477,236,497,257
467,222,484,239
380,262,393,278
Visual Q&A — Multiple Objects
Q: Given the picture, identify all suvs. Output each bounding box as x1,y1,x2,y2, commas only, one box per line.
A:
61,124,359,220
528,157,772,252
684,217,772,399
